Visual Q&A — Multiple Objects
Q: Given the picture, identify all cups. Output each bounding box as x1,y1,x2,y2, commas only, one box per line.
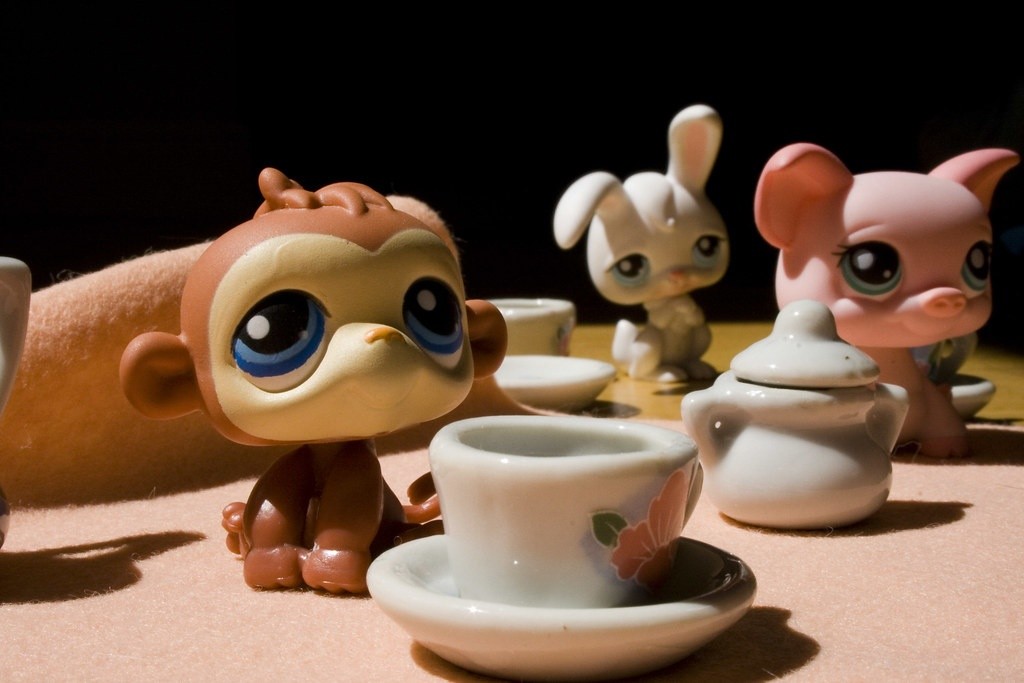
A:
483,298,576,355
425,411,705,614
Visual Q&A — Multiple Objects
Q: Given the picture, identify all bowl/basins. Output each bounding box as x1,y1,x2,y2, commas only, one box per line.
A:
679,298,911,528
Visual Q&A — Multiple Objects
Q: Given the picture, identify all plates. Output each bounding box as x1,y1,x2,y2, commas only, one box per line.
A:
930,376,999,423
494,352,617,411
367,531,758,678
